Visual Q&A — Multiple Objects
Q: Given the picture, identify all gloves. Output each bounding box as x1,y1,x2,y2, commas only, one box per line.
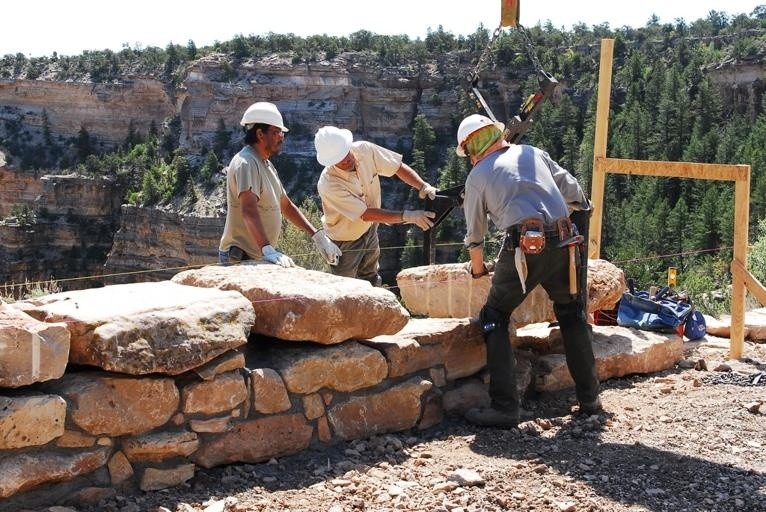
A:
262,245,295,268
403,210,436,231
311,230,342,262
419,182,441,200
464,259,496,277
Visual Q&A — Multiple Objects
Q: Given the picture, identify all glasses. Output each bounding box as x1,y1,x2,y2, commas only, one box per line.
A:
267,128,285,137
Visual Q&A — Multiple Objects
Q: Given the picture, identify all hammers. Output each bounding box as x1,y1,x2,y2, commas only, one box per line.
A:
558,235,584,295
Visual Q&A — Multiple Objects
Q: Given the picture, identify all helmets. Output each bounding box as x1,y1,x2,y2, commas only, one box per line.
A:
240,102,289,132
315,126,353,166
456,114,505,158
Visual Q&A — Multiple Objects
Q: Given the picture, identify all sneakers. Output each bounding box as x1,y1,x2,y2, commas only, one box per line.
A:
580,395,602,410
465,406,520,426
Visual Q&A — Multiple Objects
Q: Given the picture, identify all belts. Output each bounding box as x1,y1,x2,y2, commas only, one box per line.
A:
516,223,559,242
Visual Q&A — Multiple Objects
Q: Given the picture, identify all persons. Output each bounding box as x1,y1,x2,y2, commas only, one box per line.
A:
217,101,343,269
314,125,442,289
455,113,604,430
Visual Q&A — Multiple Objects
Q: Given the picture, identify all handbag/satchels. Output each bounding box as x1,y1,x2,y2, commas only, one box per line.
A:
617,291,692,333
684,311,706,339
